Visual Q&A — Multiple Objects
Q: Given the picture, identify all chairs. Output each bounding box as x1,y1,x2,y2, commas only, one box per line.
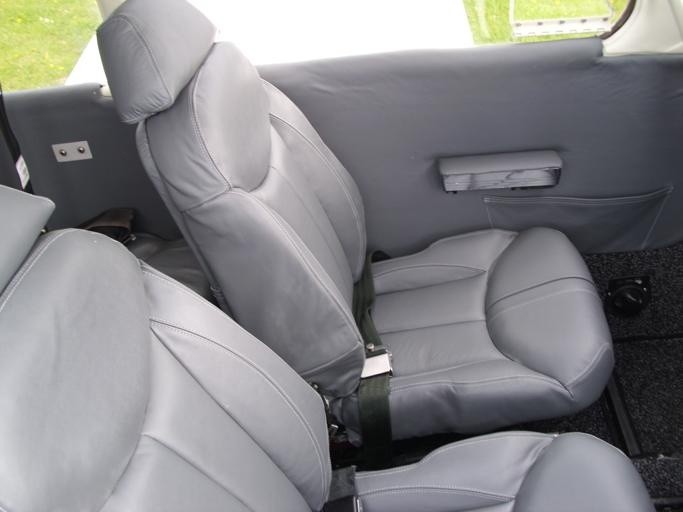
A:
0,183,654,512
96,0,618,457
